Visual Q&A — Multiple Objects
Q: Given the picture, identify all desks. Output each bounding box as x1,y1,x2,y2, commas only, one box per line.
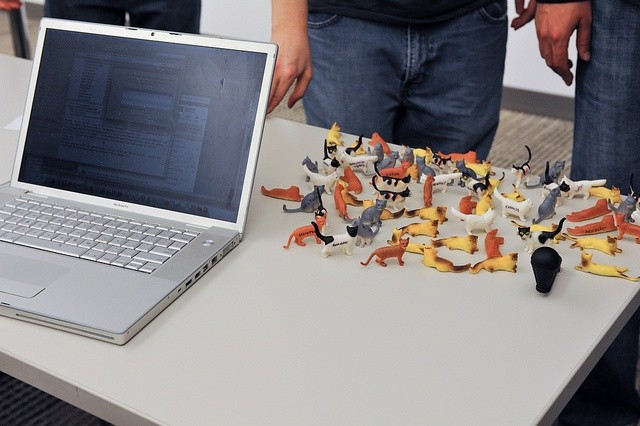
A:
1,54,638,425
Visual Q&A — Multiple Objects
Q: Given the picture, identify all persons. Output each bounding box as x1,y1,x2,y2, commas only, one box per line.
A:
267,0,538,164
44,0,201,33
533,0,639,199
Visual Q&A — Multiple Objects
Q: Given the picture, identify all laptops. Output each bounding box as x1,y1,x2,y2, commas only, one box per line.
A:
0,17,279,347
